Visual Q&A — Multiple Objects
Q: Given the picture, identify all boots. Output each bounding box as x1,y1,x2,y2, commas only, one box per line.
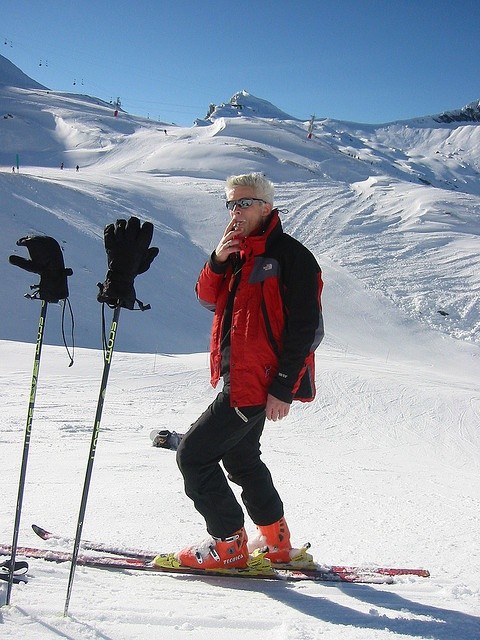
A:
176,527,249,568
247,516,293,564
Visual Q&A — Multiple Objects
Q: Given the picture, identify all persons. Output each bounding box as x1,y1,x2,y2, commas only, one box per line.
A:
175,172,326,571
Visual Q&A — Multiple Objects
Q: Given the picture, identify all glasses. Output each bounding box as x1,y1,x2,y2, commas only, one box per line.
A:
225,197,269,210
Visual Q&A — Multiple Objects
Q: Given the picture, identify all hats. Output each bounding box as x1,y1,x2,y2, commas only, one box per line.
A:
159,431,168,437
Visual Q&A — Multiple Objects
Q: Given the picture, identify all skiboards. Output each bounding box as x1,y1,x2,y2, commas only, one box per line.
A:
1,525,431,584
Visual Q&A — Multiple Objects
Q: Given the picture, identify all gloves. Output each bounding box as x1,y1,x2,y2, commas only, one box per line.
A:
9,234,73,303
95,216,159,311
